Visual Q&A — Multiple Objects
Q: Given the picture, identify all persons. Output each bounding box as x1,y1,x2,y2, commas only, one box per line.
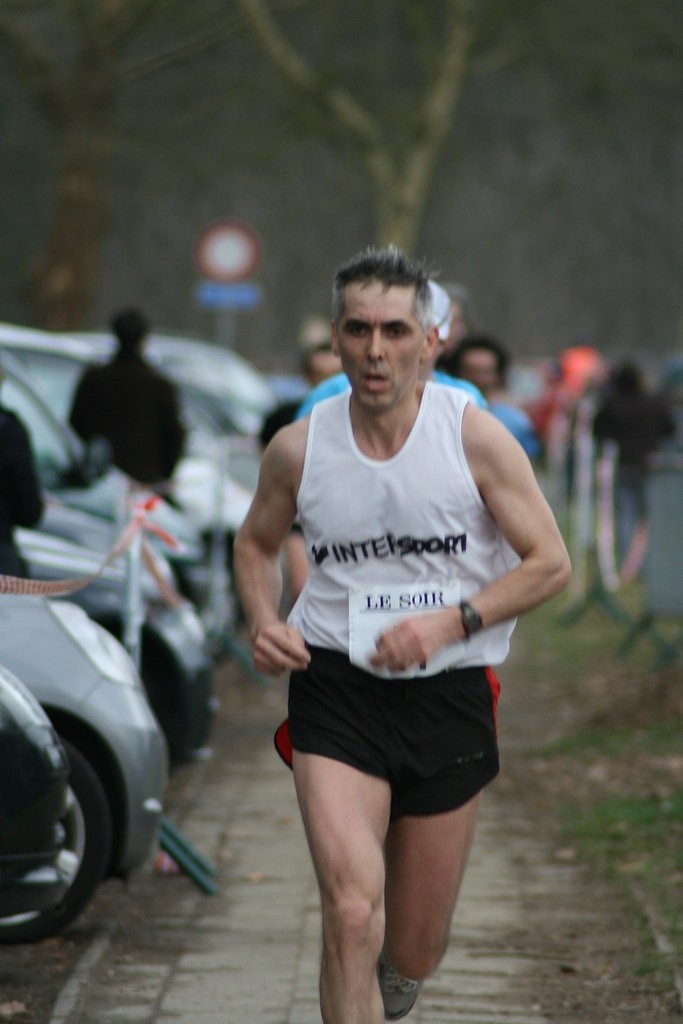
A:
0,369,45,579
258,284,673,606
71,305,189,490
236,249,570,1024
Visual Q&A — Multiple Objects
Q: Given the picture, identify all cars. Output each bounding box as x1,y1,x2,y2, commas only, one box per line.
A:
0,312,315,950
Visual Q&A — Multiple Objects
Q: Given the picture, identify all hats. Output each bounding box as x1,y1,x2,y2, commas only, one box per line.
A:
428,277,453,341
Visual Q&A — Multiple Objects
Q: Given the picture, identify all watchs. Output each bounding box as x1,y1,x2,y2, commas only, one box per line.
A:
460,601,483,640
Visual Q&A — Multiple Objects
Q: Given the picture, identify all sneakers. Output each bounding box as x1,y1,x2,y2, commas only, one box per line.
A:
376,952,423,1021
